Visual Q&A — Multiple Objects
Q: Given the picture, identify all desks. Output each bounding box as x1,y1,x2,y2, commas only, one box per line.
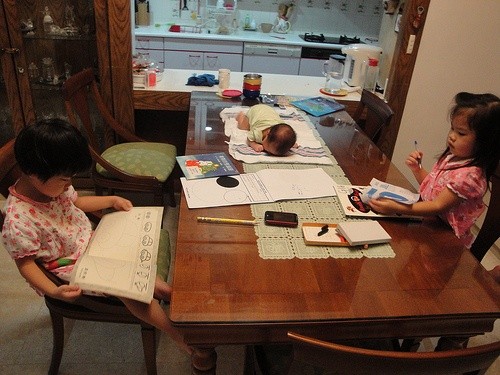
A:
168,91,500,375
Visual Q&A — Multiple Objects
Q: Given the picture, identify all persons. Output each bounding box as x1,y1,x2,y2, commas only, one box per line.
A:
368,92,500,249
0,118,219,356
236,104,298,156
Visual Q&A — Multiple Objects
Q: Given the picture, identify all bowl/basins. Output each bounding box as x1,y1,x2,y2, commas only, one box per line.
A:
243,74,262,99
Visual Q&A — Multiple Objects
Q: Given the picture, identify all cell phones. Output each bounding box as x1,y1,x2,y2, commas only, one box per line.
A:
265,210,298,226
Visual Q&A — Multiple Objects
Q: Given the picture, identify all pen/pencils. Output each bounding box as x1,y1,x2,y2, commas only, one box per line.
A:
415,140,421,169
197,217,260,226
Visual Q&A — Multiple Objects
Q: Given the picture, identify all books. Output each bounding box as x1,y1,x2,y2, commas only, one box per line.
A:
332,178,423,219
290,96,347,117
180,167,337,209
336,219,392,246
175,152,239,181
69,207,164,304
303,222,349,247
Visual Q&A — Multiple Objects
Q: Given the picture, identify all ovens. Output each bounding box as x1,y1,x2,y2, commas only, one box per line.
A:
299,43,346,76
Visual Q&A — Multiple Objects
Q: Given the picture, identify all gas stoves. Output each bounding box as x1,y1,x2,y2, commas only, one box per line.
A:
299,31,366,46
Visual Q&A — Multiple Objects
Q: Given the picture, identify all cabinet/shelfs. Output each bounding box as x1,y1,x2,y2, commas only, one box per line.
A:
134,36,163,68
0,0,134,196
164,38,243,72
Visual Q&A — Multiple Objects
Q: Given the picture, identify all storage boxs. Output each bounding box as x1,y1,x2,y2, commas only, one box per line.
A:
301,223,350,246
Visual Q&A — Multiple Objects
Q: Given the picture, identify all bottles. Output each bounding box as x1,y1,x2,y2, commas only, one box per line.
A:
149,62,156,86
21,5,91,86
232,14,257,29
170,15,203,33
362,57,379,93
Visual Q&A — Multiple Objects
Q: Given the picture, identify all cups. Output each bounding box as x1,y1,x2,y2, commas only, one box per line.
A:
219,69,230,88
41,102,55,119
261,23,276,33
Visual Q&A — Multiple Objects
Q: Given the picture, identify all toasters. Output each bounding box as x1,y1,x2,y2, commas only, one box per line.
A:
345,43,383,87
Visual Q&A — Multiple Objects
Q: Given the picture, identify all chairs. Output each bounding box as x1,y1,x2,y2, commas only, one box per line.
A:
63,68,175,208
0,136,158,375
244,332,500,375
469,165,500,263
354,90,395,153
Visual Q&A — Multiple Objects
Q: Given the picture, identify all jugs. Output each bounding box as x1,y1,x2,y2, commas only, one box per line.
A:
322,55,345,93
